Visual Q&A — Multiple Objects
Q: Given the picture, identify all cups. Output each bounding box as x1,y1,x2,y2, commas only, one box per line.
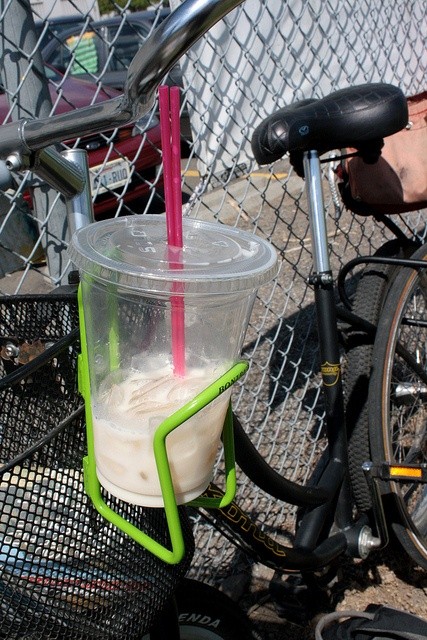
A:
67,214,282,510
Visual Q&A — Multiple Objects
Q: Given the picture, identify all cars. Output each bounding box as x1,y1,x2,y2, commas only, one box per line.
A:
38,6,192,139
1,54,169,216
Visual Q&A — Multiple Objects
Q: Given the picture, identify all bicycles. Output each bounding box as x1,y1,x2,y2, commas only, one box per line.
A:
0,1,425,640
